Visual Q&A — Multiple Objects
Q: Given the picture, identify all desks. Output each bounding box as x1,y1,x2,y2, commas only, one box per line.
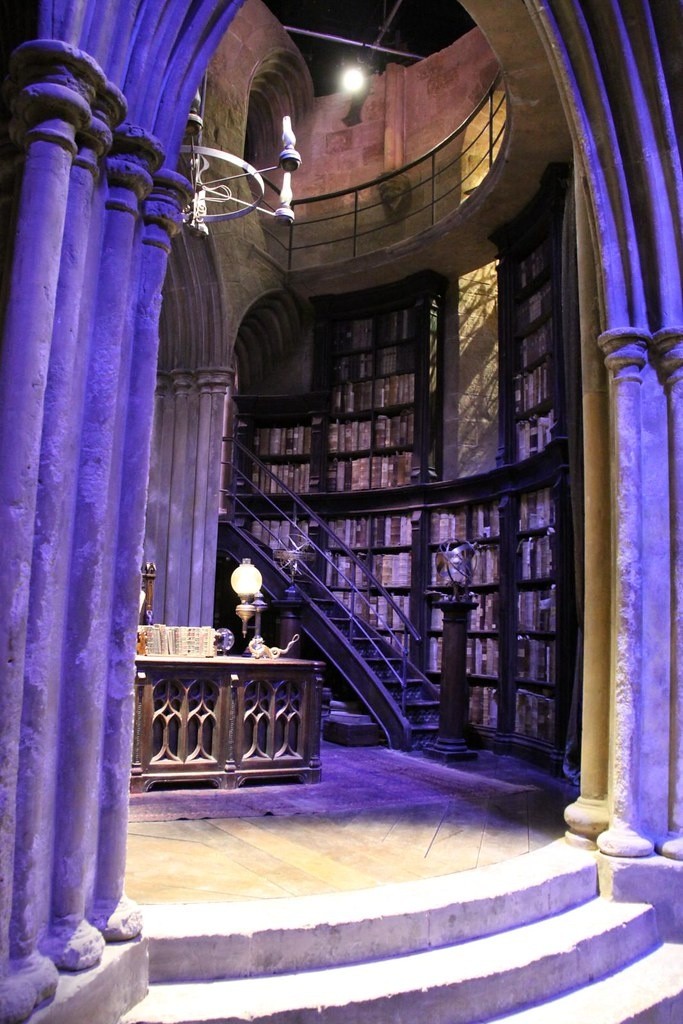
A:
129,655,323,794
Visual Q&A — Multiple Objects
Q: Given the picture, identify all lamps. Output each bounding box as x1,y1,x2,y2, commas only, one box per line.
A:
231,557,262,638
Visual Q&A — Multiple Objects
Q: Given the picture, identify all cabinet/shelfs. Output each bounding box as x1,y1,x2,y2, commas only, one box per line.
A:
231,269,450,496
236,463,579,778
488,159,573,468
